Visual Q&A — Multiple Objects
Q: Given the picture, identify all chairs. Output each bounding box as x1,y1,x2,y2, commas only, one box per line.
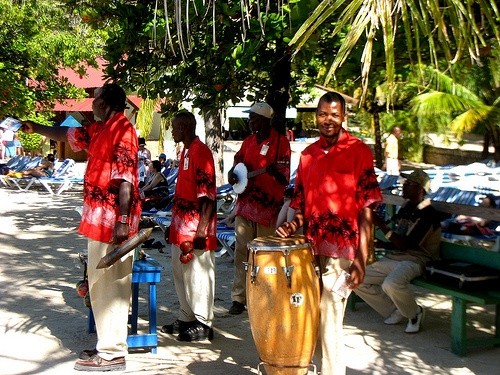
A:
0,156,44,188
431,184,460,202
377,172,400,190
39,176,86,195
454,187,480,205
142,159,235,260
0,155,22,169
8,157,75,191
0,155,31,175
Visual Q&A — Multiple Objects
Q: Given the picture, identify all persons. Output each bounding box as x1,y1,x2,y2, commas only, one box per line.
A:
352,170,442,334
275,92,385,375
0,123,20,162
72,83,142,371
137,137,169,200
218,205,236,228
380,126,403,177
458,195,496,225
226,103,291,314
160,110,217,343
276,187,297,226
9,154,57,178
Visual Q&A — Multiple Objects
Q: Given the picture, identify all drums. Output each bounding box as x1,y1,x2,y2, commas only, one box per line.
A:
245,233,321,375
232,155,291,195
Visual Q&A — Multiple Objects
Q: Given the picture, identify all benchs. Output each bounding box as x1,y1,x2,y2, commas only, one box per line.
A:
349,190,500,361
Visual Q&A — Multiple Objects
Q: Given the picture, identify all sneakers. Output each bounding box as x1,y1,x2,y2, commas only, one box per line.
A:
161,319,197,334
177,325,214,341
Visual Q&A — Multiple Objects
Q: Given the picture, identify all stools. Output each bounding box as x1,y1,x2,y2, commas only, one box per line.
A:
80,253,162,356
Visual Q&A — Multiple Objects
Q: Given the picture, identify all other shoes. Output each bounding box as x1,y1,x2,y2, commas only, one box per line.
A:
402,304,424,333
229,302,244,314
79,348,98,360
141,238,165,249
74,355,126,371
384,310,402,325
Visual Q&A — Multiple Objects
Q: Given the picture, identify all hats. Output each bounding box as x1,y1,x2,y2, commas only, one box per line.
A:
138,138,145,145
159,153,166,158
401,168,431,195
242,102,275,119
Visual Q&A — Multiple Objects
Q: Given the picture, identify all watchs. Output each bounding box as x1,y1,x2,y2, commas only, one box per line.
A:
118,213,132,224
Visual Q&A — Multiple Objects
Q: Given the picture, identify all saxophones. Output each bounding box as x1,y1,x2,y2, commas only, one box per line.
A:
366,185,399,265
